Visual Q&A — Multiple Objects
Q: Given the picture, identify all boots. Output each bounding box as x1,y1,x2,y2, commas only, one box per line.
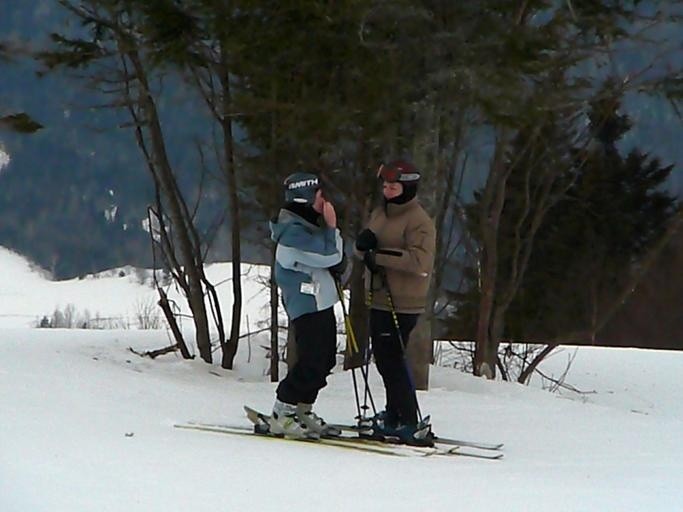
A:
268,397,342,440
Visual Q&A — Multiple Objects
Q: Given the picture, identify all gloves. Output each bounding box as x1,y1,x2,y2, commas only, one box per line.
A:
355,228,378,251
363,250,385,274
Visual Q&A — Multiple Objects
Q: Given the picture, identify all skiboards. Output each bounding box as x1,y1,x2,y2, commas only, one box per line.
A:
174,419,460,458
173,406,504,459
328,423,504,458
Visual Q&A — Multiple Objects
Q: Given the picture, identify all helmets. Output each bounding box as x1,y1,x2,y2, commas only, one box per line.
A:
376,158,421,193
282,172,321,206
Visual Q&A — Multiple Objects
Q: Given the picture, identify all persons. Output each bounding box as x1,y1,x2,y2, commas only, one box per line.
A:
265,172,341,442
351,159,433,440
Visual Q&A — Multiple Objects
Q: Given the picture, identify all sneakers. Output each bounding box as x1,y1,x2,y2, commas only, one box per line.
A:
356,409,435,448
